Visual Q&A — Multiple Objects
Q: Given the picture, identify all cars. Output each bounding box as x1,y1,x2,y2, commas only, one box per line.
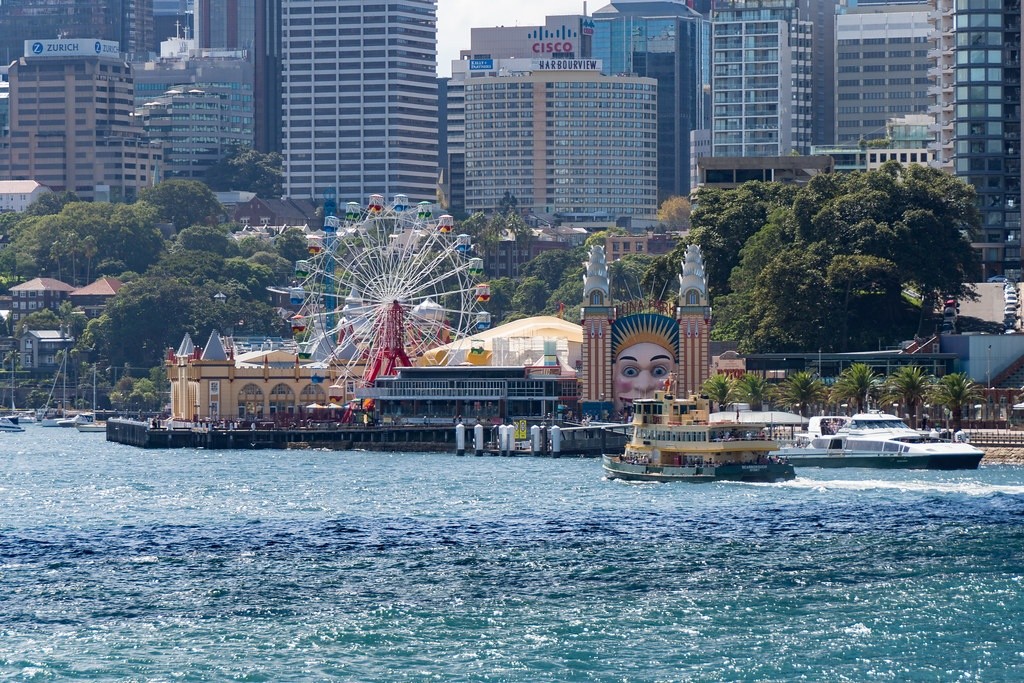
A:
938,273,1019,337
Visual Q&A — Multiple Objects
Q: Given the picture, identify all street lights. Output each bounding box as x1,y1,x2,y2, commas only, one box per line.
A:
987,344,993,389
818,346,822,380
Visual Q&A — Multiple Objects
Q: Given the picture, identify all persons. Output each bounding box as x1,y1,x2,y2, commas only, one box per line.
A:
619,419,971,468
143,403,630,433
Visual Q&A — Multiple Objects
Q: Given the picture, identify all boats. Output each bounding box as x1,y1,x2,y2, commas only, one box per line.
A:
602,371,798,483
769,408,987,470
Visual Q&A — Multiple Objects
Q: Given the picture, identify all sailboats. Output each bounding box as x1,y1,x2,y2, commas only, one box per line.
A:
0,347,107,432
